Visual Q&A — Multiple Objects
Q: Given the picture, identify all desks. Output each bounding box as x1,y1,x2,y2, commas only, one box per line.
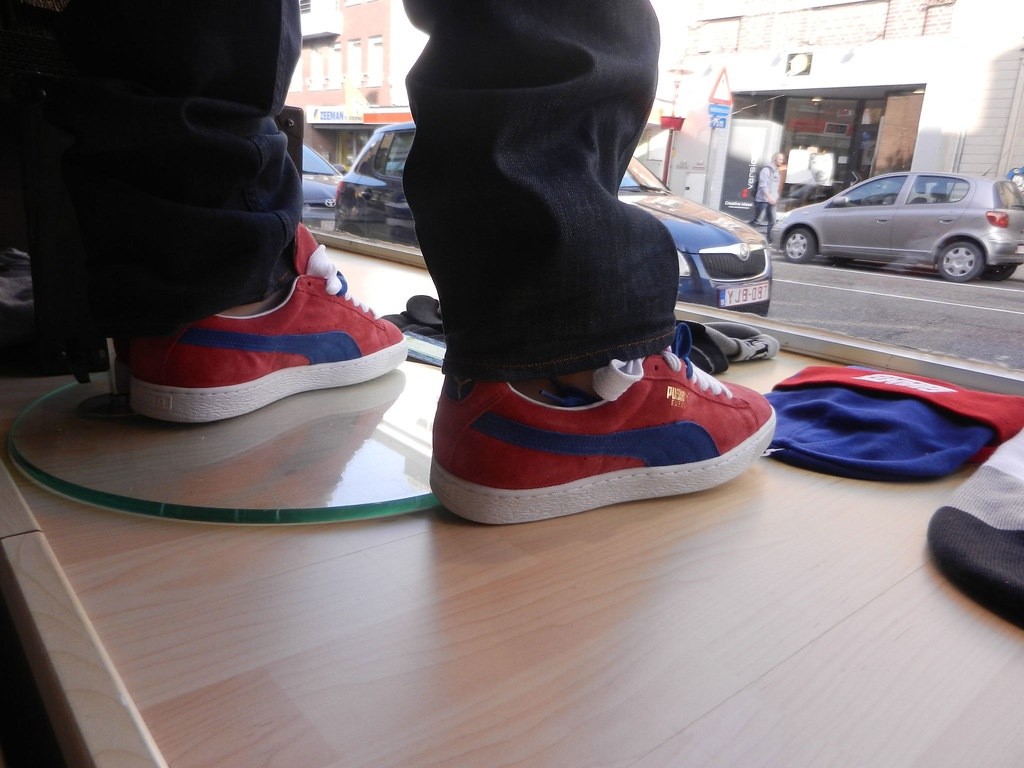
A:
0,224,1024,767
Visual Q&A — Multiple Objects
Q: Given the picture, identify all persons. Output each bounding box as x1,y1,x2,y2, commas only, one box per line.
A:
77,0,776,529
748,152,785,247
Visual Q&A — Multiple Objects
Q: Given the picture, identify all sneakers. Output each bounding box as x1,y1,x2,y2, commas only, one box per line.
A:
431,352,776,524
112,222,407,423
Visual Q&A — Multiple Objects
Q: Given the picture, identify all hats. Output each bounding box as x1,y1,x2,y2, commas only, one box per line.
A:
929,427,1024,630
676,320,779,376
762,366,1022,480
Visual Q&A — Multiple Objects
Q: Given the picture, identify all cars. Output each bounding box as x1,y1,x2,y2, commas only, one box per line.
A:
336,122,770,315
300,141,346,229
769,171,1024,282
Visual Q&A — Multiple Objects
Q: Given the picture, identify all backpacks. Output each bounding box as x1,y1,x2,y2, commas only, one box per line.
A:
750,166,774,197
